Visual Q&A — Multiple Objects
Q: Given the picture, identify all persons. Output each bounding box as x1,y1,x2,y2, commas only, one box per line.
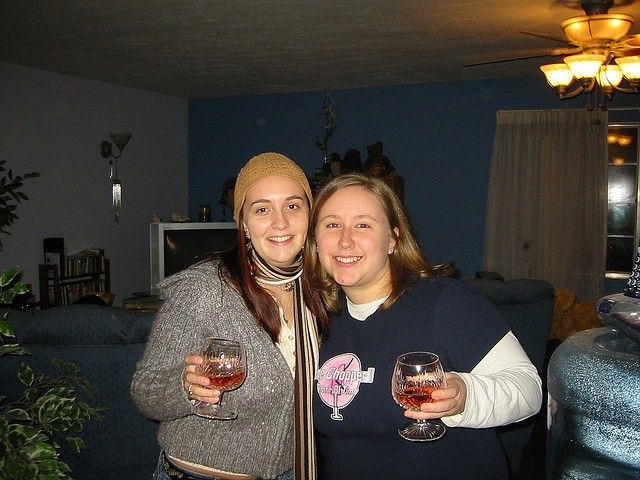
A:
131,153,314,479
302,175,543,479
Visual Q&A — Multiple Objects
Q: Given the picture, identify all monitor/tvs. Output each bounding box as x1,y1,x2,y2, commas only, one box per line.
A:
150,222,241,294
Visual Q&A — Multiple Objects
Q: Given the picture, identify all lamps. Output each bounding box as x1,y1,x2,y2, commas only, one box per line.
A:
563,54,607,93
595,65,624,100
560,13,635,46
615,54,640,94
99,132,133,222
607,127,632,165
540,63,574,100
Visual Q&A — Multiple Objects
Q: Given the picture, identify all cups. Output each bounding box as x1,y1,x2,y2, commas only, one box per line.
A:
195,336,248,422
390,350,448,442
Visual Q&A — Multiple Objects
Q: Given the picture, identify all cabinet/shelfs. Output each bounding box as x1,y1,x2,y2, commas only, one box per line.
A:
39,248,110,310
305,173,405,209
124,296,165,316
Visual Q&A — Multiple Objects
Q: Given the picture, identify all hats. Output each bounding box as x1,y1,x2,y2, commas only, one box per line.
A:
233,152,314,230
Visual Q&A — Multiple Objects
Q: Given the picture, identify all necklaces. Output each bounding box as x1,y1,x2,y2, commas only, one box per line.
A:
252,268,304,292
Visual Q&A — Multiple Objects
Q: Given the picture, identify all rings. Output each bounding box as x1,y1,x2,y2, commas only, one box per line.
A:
188,384,193,396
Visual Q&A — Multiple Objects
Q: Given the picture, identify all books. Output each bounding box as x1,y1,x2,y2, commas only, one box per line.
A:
64,255,106,276
61,280,105,306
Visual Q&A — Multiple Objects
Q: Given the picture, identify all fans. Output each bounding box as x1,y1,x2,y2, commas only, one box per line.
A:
462,0,640,68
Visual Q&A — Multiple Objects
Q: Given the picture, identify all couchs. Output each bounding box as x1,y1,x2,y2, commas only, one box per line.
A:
0,306,161,479
462,277,553,479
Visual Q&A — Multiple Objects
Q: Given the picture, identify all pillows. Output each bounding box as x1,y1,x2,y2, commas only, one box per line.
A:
551,288,578,320
